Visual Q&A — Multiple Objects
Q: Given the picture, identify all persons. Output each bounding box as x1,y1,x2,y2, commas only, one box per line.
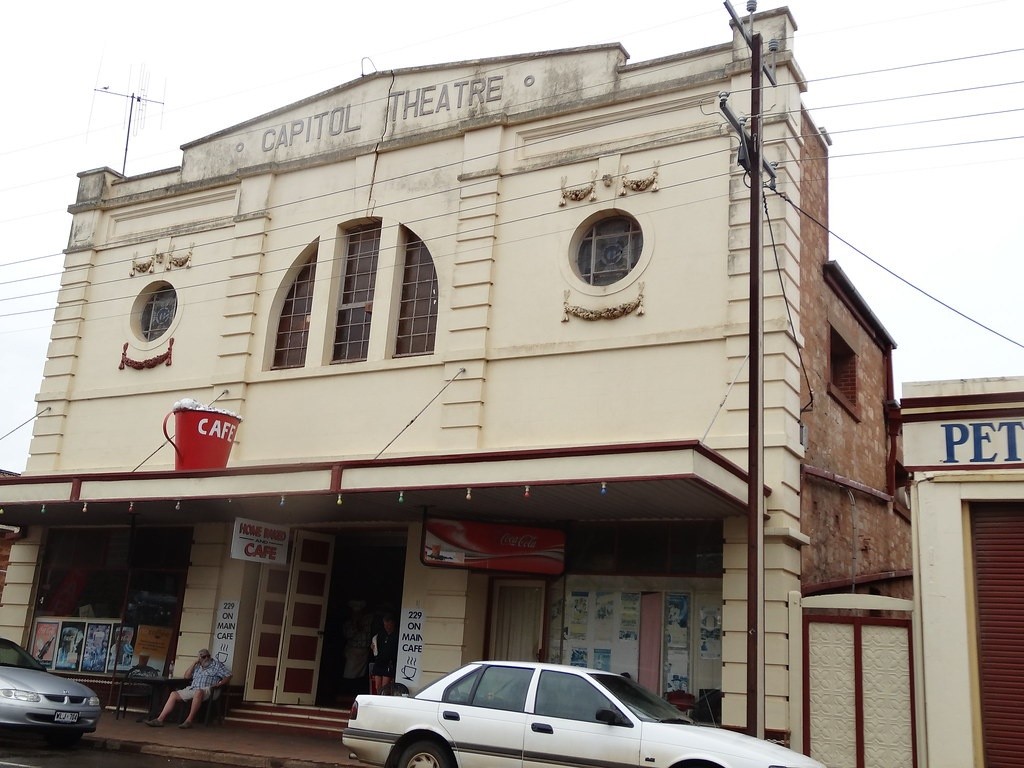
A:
371,612,398,692
145,649,232,728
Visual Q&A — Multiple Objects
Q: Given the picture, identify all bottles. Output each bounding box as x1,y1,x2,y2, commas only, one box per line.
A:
38,635,55,660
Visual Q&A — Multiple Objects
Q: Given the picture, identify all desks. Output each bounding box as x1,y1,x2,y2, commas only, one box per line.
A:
141,677,190,722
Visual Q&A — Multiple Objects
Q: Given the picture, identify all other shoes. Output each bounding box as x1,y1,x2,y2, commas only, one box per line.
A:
145,718,164,726
179,721,193,729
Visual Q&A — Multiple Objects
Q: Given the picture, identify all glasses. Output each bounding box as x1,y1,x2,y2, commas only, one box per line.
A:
200,654,210,658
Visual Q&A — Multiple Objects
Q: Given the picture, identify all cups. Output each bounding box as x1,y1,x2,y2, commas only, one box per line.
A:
139,652,152,667
164,405,243,471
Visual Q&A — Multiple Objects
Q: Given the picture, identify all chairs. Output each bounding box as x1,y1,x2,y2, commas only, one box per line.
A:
115,663,158,723
181,683,229,727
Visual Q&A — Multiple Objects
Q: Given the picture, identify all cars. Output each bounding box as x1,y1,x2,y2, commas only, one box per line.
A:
0,637,102,750
342,658,828,768
